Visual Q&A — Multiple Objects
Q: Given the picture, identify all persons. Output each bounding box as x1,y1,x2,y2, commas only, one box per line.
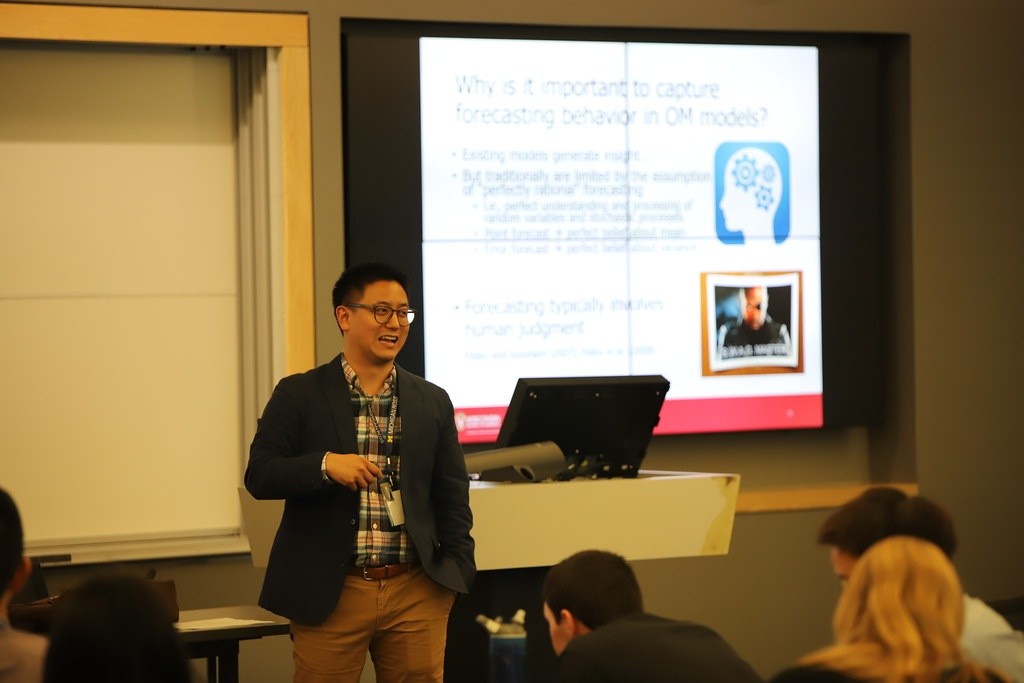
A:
818,486,1024,682
0,491,49,682
542,549,768,683
244,260,478,683
768,534,1016,683
717,287,790,358
41,576,194,682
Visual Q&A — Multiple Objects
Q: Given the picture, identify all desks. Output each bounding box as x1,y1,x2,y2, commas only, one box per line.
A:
175,606,290,683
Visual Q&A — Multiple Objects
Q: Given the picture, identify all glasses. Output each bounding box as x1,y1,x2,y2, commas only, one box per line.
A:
345,303,417,325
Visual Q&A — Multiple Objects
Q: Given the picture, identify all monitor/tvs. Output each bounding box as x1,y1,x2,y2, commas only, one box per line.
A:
479,376,668,480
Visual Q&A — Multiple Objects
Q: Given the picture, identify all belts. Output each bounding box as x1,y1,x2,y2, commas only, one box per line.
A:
347,564,414,579
